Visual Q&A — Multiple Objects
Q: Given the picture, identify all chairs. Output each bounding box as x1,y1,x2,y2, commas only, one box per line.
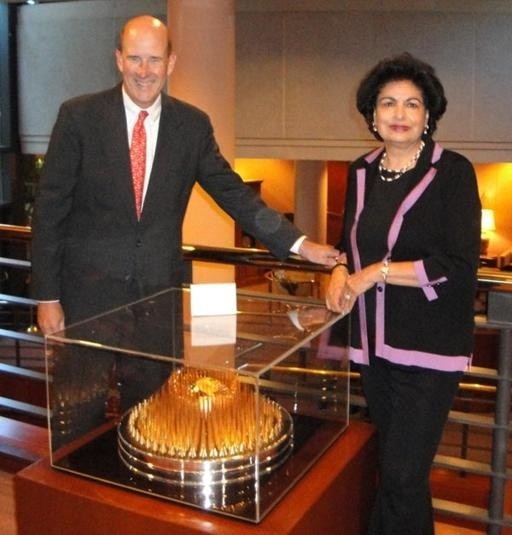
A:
500,250,512,271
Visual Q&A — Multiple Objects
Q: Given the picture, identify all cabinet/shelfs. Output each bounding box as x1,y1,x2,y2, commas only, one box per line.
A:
15,290,376,535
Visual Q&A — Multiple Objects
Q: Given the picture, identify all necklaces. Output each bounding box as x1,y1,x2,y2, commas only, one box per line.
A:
377,141,425,182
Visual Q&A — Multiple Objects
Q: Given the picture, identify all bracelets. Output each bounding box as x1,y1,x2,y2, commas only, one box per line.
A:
382,260,388,284
329,262,349,274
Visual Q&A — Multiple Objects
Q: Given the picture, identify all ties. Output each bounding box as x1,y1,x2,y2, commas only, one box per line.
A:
130,111,149,222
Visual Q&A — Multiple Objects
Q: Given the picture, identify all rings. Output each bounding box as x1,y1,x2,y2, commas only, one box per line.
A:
338,249,344,258
344,292,351,301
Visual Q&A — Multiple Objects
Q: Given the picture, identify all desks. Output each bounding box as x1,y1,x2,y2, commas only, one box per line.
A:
266,269,323,327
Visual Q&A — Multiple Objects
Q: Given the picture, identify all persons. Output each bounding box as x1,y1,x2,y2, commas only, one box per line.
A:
30,14,346,450
325,51,482,535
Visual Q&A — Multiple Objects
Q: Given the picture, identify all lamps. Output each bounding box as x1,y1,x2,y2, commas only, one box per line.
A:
479,210,495,256
